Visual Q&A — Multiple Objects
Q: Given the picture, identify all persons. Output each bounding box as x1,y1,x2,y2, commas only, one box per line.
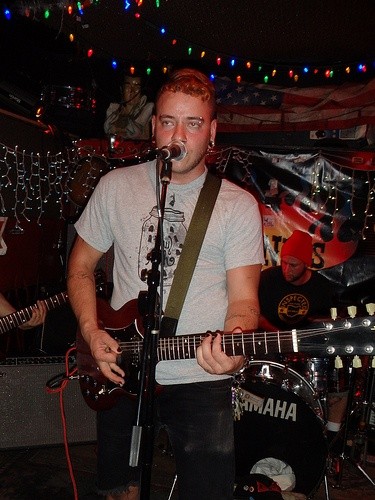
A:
104,70,153,139
67,68,266,500
258,229,351,456
0,292,46,330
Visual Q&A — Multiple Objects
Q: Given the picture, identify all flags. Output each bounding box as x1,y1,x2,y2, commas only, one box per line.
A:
212,77,375,133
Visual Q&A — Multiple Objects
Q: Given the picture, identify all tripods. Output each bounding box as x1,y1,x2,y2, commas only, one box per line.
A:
328,357,375,490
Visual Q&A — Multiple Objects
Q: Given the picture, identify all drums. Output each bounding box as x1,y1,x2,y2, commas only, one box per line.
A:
321,356,350,397
230,360,328,495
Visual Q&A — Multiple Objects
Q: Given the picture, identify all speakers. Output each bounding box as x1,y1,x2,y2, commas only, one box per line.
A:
0,351,96,452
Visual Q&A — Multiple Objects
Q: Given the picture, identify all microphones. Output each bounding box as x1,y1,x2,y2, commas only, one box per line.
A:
156,140,187,162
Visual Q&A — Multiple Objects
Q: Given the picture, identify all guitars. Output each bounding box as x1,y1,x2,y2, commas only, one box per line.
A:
77,295,375,414
0,273,104,335
